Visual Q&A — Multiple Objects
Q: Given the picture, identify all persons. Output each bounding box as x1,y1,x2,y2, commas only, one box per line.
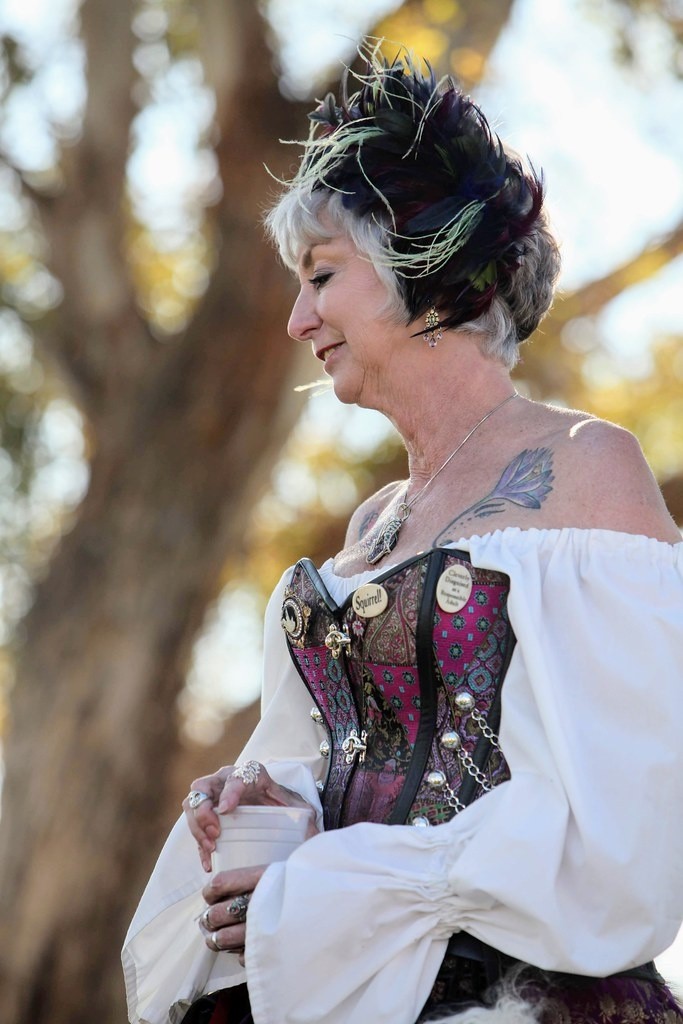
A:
120,35,683,1024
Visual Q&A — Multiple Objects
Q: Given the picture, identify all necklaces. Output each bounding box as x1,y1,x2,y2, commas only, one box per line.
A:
365,393,518,565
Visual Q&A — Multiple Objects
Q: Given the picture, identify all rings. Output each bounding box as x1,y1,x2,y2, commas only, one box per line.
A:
227,894,250,923
202,905,225,953
188,790,208,809
232,760,260,785
